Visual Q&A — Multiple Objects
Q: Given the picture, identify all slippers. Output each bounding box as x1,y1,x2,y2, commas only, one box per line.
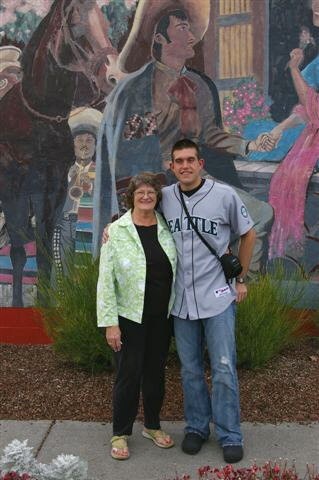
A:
110,434,130,460
142,429,175,449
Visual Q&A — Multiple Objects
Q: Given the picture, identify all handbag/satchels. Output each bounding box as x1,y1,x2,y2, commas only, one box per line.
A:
219,254,243,286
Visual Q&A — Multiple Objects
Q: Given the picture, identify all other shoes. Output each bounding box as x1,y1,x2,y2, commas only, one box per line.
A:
181,433,203,455
222,446,243,464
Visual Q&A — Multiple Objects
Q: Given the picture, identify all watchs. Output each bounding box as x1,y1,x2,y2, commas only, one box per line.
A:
237,278,245,283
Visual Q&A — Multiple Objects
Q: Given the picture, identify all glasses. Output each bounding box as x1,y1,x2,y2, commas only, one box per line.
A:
132,190,159,197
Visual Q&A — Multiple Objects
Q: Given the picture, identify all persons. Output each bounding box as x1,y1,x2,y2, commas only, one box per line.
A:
102,139,257,464
96,171,178,459
